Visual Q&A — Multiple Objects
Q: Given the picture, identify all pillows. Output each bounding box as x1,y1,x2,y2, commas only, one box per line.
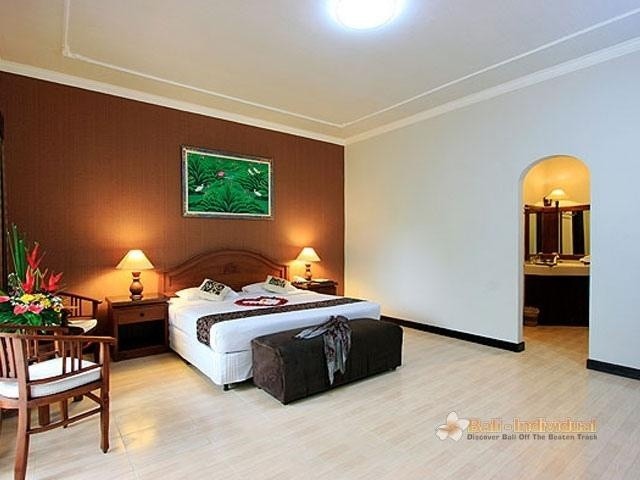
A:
175,287,239,301
261,274,290,294
243,281,293,293
196,278,231,301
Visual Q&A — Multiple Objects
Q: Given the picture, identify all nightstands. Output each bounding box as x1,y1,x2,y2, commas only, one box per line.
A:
292,281,338,295
105,292,169,361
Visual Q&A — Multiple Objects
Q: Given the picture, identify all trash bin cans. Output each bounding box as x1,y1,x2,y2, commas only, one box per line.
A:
524,306,540,327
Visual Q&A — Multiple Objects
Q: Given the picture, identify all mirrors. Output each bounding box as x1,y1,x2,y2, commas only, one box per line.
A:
524,204,591,260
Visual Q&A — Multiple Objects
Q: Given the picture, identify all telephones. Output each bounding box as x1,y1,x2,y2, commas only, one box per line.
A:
293,276,308,284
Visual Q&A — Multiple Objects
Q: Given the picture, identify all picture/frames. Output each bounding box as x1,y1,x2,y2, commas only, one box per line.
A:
181,143,275,221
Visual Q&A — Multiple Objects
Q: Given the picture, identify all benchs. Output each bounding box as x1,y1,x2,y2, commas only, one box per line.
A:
251,319,403,404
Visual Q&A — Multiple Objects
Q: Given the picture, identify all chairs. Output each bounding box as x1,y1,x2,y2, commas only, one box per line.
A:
52,290,103,363
0,333,116,480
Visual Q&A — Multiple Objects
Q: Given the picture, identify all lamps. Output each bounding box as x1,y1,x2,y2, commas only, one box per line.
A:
116,250,155,300
544,188,569,208
296,247,321,284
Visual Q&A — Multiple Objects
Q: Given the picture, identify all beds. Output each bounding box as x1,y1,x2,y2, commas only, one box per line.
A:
157,249,381,392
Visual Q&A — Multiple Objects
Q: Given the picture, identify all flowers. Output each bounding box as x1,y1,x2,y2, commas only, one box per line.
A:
0,222,79,336
